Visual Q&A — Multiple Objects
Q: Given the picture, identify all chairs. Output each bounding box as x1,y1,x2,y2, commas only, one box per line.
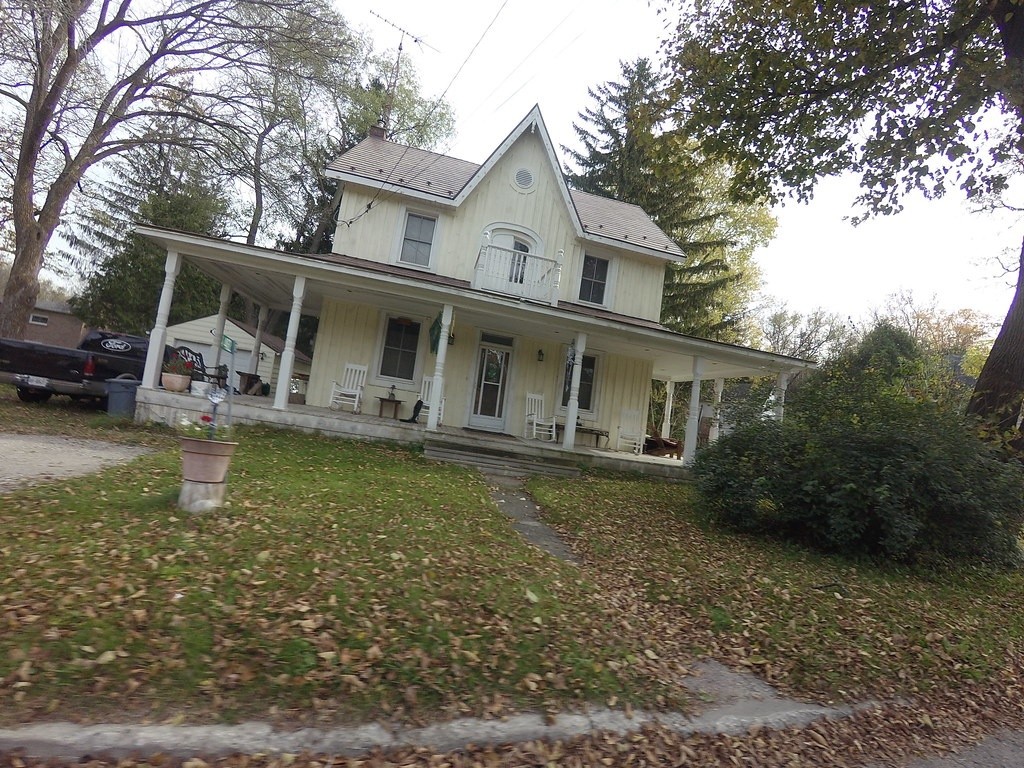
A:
326,360,368,415
416,374,447,427
615,409,645,455
524,390,557,443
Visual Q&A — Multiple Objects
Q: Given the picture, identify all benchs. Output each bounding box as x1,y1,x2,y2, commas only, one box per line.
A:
165,344,228,393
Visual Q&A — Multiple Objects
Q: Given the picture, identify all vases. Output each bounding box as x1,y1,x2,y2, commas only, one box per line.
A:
190,380,213,399
388,391,395,400
576,421,582,426
178,435,240,483
161,372,191,392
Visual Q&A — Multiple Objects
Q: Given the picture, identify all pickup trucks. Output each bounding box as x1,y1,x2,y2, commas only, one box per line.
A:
0,326,186,418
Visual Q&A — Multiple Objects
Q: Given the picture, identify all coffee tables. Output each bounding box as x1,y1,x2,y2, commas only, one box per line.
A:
374,396,407,419
548,421,610,451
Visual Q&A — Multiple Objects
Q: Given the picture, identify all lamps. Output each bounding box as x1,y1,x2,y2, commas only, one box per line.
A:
536,349,544,361
447,333,454,345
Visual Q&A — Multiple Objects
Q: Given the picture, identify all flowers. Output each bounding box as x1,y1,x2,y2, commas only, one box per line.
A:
161,352,193,375
566,347,575,366
179,412,230,441
390,384,397,393
577,416,580,422
